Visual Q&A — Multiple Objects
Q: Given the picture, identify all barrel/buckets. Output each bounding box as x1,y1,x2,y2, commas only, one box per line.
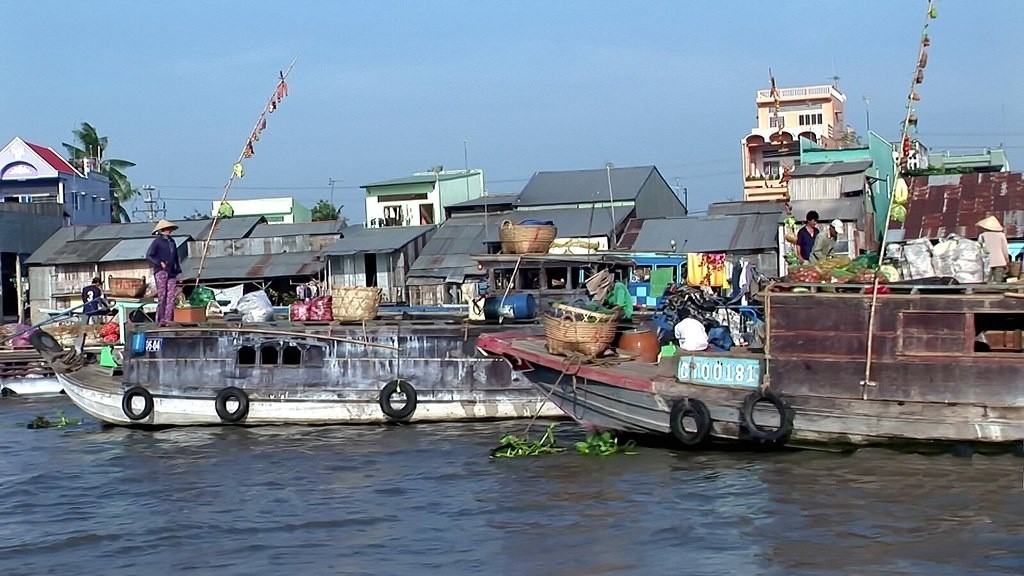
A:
484,294,536,319
132,334,146,353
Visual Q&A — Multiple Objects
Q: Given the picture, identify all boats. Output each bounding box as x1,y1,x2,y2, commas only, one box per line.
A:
29,255,636,430
480,273,1023,455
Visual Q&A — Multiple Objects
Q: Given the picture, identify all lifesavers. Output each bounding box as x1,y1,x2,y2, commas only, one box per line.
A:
122,386,153,419
740,389,795,441
670,398,711,446
380,381,418,418
215,386,250,420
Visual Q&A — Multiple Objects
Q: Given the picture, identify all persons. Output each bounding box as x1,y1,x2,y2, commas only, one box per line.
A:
81,277,107,326
146,219,181,326
974,215,1010,285
809,219,843,263
797,210,820,265
594,270,634,324
673,308,711,351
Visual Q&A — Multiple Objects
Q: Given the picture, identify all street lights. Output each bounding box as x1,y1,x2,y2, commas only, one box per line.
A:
605,162,620,245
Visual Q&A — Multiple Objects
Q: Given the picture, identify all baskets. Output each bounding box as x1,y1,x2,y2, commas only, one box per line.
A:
108,274,146,298
499,219,558,254
545,303,621,359
331,285,382,324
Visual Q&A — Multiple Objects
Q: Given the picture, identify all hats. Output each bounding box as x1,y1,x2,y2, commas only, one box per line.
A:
93,277,102,286
975,215,1004,232
831,219,843,235
152,219,179,235
